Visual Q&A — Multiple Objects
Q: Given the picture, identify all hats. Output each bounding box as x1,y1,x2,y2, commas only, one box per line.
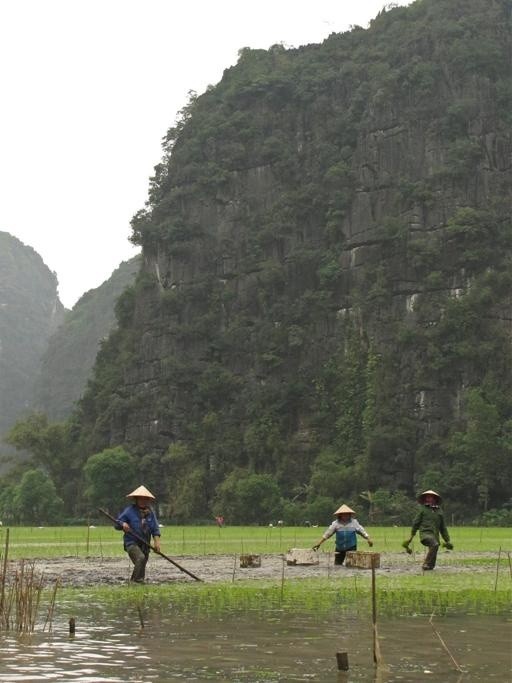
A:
125,485,156,499
333,503,355,515
418,490,442,505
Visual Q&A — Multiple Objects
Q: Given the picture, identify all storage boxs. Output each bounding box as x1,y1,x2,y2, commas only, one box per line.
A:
287,548,320,565
239,554,261,567
346,551,380,569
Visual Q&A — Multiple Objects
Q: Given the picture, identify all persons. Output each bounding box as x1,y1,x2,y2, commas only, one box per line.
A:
312,504,372,565
114,485,160,584
408,489,450,569
